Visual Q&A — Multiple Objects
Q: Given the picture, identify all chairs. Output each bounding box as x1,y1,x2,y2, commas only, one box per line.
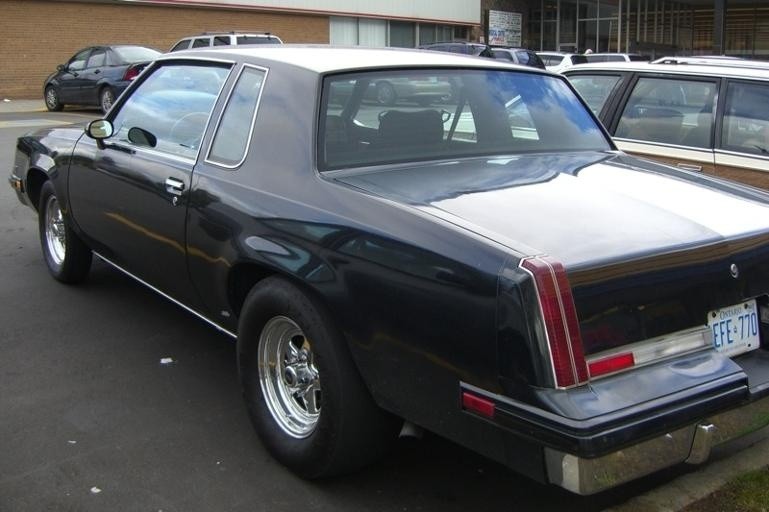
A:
319,106,445,166
636,103,730,148
